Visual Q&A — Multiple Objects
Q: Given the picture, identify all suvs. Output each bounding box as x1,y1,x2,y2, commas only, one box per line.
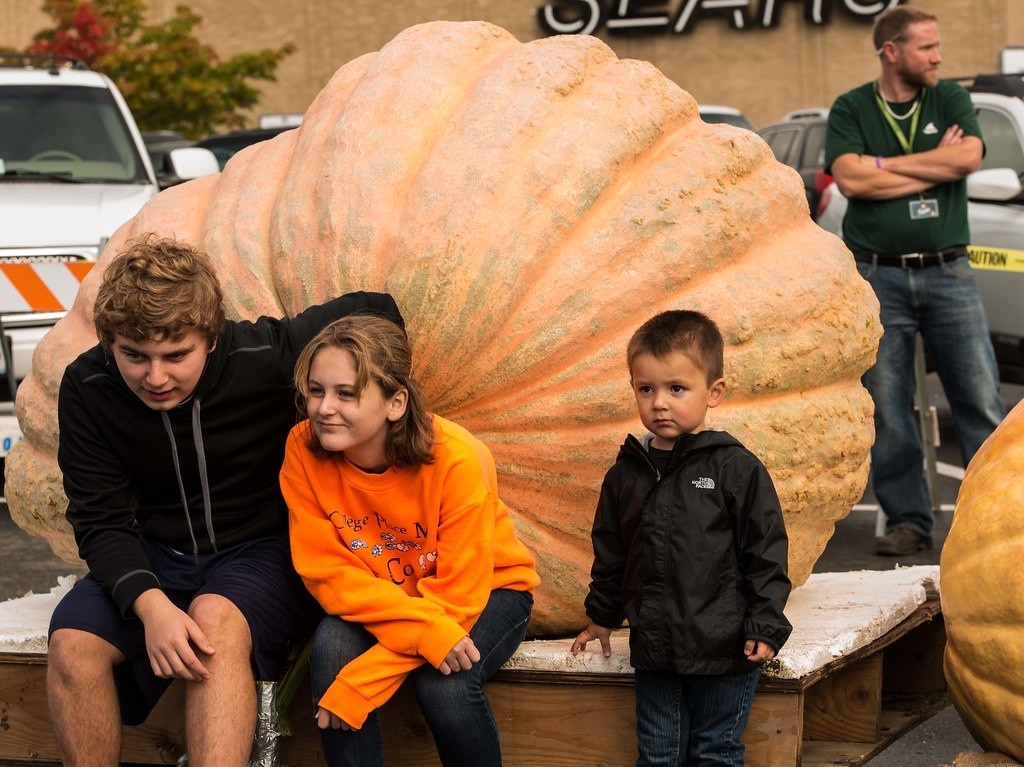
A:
0,54,223,462
752,72,1024,390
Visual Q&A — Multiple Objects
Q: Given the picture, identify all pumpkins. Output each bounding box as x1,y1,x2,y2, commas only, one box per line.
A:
5,22,882,637
939,393,1024,767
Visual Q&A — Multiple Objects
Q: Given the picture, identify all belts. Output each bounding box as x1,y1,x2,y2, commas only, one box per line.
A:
852,246,967,271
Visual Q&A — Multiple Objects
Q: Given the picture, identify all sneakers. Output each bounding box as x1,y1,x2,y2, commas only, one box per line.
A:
875,527,934,556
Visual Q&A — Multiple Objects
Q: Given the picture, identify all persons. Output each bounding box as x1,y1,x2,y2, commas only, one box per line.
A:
571,311,794,767
917,204,931,216
46,232,408,767
824,8,1006,557
278,318,542,767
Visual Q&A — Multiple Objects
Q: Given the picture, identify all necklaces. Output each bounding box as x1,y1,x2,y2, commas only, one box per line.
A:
879,81,918,120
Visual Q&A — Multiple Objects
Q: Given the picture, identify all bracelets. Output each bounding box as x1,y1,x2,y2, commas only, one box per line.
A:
876,155,881,167
